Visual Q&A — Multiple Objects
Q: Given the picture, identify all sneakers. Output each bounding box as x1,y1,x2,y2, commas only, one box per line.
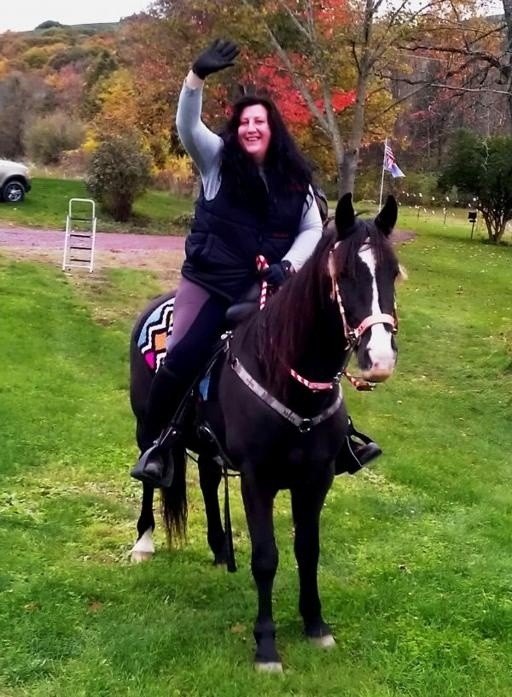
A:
131,453,171,487
336,438,381,475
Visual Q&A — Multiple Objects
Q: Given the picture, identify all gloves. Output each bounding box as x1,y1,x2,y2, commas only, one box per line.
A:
193,37,239,81
254,261,291,292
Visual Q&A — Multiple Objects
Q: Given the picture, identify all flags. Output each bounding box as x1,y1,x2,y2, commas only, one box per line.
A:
384,144,406,180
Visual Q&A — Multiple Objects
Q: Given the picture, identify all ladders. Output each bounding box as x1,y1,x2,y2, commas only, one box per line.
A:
62,198,97,273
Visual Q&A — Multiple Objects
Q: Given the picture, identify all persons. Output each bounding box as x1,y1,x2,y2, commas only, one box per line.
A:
132,36,381,487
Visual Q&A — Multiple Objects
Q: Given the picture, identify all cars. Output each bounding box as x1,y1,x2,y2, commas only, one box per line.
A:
1,160,33,201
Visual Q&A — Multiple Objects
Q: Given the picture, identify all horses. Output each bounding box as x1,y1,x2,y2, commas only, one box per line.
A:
129,193,409,679
